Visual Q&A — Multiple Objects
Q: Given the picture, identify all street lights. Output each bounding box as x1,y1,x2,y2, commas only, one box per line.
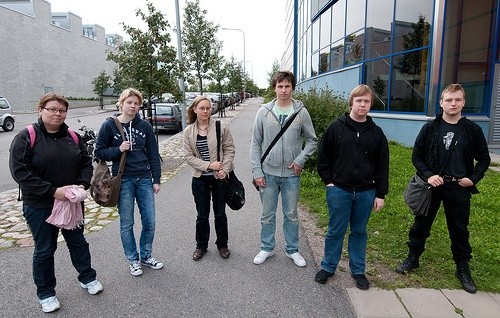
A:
222,27,246,101
241,60,253,98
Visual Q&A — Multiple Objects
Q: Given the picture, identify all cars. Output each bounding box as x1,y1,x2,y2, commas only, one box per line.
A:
0,96,16,132
206,91,252,115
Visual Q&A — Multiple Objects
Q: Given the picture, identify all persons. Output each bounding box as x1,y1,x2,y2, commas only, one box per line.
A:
9,94,103,312
317,85,390,289
397,84,491,293
94,88,163,275
250,71,318,267
183,96,236,261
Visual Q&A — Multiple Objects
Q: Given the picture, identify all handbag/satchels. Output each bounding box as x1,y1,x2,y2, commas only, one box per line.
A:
90,162,122,207
404,175,433,217
223,171,246,211
253,172,260,191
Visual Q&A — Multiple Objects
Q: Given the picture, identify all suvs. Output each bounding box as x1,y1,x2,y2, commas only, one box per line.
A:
144,97,183,135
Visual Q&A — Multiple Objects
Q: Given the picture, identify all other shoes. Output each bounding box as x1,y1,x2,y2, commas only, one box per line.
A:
193,249,208,260
218,246,229,259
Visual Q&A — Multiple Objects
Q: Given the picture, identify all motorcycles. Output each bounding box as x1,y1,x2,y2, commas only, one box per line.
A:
77,117,98,162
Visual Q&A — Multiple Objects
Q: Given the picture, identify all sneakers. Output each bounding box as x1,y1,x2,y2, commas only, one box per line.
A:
316,270,335,284
81,280,104,294
396,259,419,273
252,250,275,265
130,261,143,276
455,271,477,293
284,250,306,266
141,258,163,269
351,273,370,290
39,296,60,312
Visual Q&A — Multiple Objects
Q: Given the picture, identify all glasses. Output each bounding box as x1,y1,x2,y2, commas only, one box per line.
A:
196,106,209,111
44,107,67,113
